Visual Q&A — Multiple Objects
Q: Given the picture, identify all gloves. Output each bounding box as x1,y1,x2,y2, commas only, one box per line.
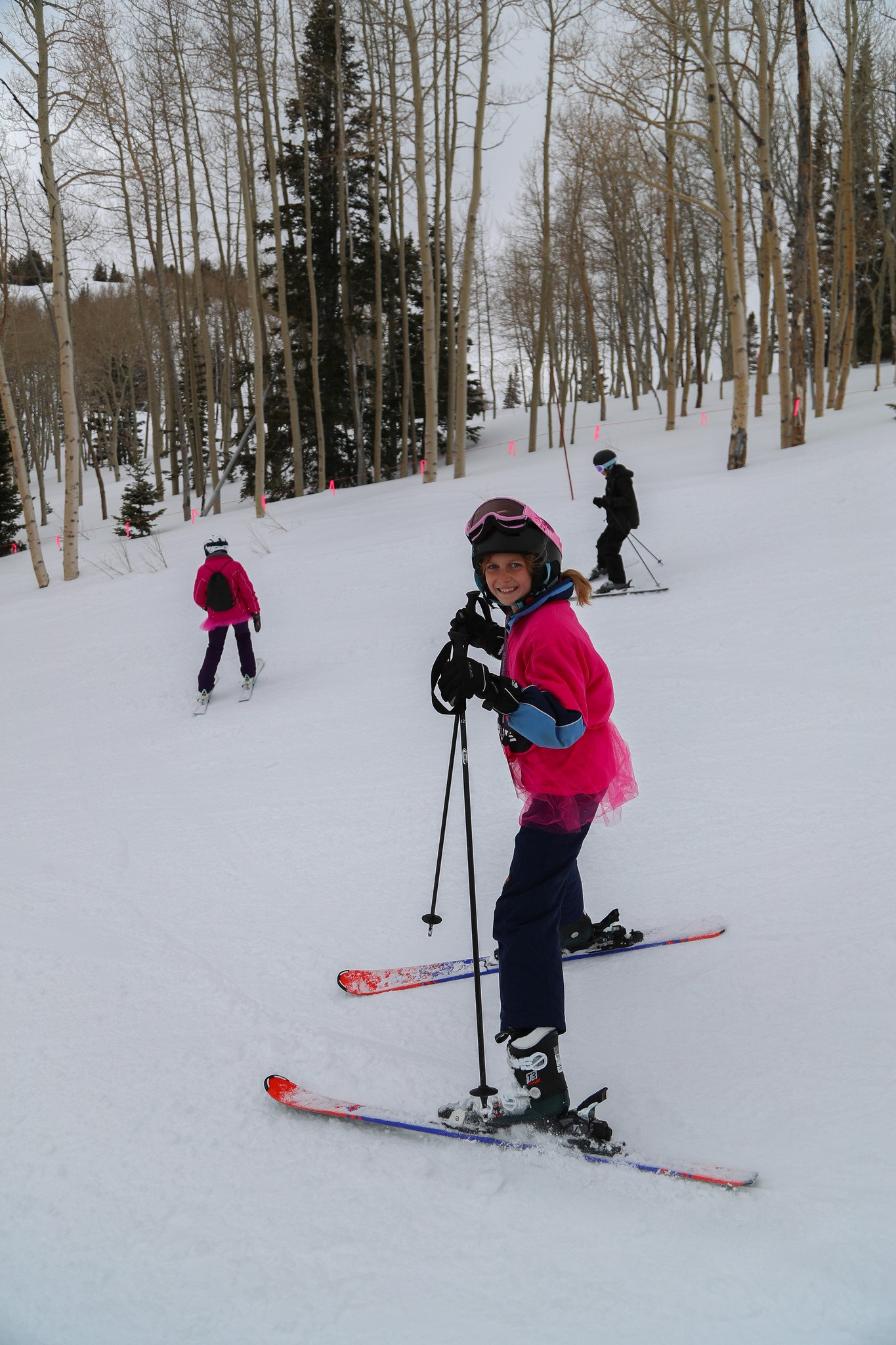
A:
450,608,500,652
438,656,490,703
251,613,261,632
593,497,604,508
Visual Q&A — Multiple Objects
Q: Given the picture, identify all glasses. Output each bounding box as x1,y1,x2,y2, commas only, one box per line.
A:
465,497,529,544
596,465,604,474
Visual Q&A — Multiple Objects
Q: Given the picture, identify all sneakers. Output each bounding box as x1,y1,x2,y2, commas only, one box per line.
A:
591,565,608,577
600,580,626,591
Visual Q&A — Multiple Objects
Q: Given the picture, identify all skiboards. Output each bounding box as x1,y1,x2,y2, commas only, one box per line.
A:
569,557,670,599
188,658,265,718
263,914,761,1187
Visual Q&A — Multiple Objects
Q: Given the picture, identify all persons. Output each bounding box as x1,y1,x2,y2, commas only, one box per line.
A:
194,534,261,704
586,449,640,593
436,497,638,1128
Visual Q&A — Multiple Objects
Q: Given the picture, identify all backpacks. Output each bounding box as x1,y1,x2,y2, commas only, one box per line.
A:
202,560,239,611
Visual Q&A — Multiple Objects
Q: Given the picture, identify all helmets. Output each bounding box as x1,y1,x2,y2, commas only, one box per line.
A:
472,518,562,609
593,450,617,471
204,533,228,557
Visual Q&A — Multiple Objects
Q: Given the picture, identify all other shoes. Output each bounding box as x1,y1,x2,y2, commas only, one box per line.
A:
560,913,593,949
439,1027,570,1128
